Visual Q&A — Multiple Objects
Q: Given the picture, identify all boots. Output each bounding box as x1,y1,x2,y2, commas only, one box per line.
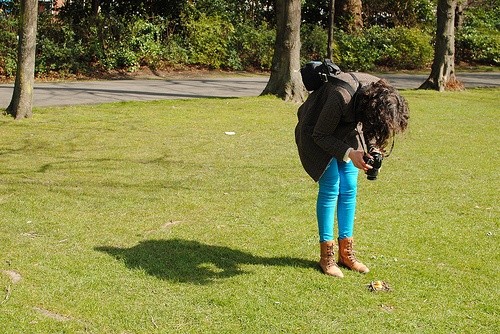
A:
320,240,345,278
336,237,370,274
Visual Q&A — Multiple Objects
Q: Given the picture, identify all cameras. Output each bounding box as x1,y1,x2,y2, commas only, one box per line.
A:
363,152,383,180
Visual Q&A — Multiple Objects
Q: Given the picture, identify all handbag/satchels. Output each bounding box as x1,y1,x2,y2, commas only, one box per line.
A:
301,60,360,97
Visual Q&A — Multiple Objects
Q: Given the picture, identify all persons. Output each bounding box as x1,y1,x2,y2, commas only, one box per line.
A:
295,73,410,278
